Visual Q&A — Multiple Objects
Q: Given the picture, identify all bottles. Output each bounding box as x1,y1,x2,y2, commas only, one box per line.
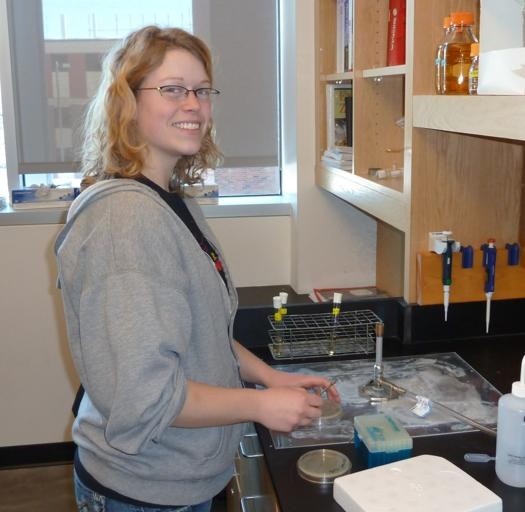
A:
468,40,478,95
443,13,474,94
435,17,450,93
497,383,524,487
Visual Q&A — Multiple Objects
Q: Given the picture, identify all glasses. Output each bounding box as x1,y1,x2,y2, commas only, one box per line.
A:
132,84,222,103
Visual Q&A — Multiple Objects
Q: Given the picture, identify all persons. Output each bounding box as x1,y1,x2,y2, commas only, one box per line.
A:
53,26,340,512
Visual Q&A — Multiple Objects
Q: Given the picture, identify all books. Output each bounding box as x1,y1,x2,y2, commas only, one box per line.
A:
321,145,353,170
336,0,354,72
386,0,406,67
326,83,353,151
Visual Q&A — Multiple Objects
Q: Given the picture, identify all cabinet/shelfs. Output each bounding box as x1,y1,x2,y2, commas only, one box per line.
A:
312,0,481,234
